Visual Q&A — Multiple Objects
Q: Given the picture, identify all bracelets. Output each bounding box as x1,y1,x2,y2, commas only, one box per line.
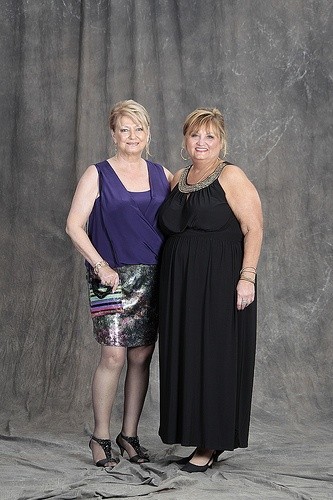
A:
93,260,109,274
239,267,256,274
239,274,255,284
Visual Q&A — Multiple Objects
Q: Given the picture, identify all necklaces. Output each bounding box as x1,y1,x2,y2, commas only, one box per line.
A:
197,159,218,181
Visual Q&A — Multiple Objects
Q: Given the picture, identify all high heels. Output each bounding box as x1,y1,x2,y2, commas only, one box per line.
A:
180,449,216,473
89,435,118,469
168,450,224,465
115,431,150,463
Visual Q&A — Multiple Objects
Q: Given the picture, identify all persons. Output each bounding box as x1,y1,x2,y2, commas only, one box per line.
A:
155,107,263,470
66,98,175,465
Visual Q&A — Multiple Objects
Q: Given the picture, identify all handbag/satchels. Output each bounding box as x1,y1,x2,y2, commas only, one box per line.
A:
90,284,124,317
